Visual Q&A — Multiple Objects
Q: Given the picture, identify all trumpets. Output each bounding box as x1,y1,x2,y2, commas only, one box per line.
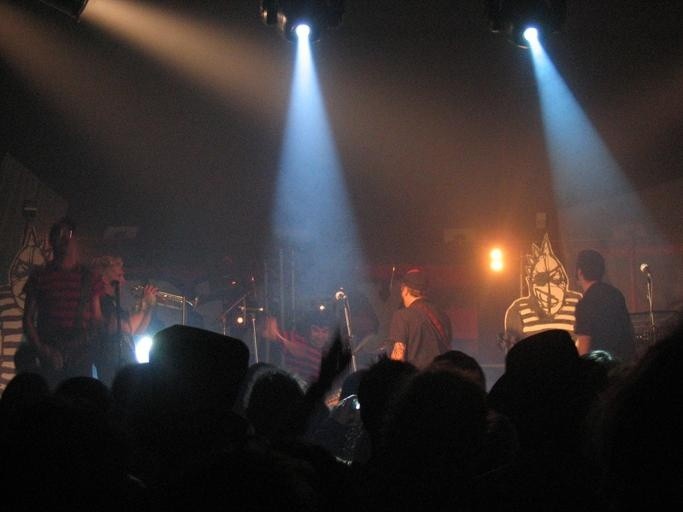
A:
123,283,199,311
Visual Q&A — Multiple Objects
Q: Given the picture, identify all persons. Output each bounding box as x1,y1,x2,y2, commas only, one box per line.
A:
571,248,639,364
388,268,451,374
3,308,682,512
89,249,162,387
21,214,120,389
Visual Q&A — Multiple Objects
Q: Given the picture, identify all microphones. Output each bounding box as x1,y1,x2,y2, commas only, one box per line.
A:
335,292,347,306
640,262,654,282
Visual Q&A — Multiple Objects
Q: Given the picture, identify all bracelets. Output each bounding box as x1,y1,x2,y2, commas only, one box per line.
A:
137,303,152,313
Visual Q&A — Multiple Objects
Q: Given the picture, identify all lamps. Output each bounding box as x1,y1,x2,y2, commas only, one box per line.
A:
258,0,343,46
479,0,568,47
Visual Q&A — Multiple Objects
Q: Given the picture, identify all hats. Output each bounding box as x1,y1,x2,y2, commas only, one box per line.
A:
405,266,425,288
